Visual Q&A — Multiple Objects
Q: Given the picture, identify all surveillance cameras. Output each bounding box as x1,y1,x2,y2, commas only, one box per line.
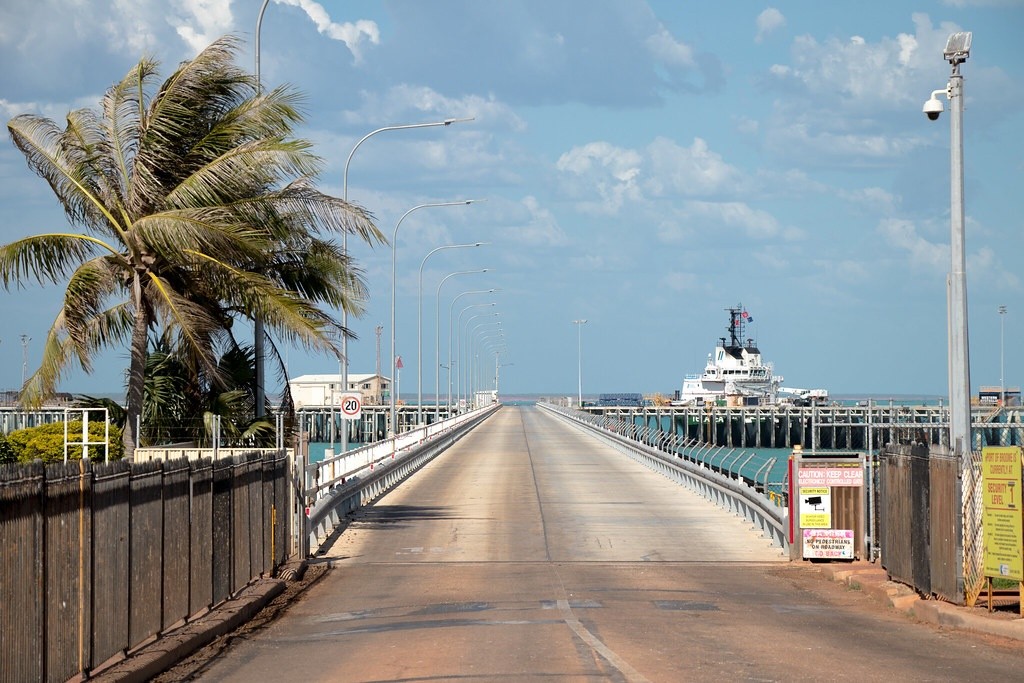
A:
922,100,944,121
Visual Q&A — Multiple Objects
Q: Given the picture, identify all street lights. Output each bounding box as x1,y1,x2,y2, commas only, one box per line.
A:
998,304,1008,408
340,113,478,456
417,240,510,430
18,330,33,388
921,76,975,464
571,320,587,407
389,191,484,437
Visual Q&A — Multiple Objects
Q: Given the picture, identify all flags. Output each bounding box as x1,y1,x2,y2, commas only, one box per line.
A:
735,320,740,325
747,316,754,323
742,312,749,318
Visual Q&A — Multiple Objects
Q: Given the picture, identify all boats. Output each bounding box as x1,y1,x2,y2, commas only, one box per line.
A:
669,306,829,424
